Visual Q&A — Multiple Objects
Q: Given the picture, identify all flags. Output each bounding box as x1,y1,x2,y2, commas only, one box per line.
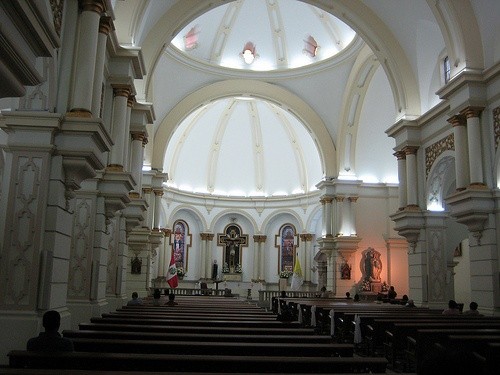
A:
291,257,304,291
166,255,178,288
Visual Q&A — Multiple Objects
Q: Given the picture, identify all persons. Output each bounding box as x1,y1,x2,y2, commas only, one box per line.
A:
345,292,352,299
320,287,326,298
127,292,143,306
212,282,223,296
212,259,218,280
279,290,289,298
27,310,73,352
401,295,409,305
406,300,416,311
443,300,458,316
463,302,479,315
370,254,380,279
354,293,360,302
149,292,161,304
388,286,397,299
165,293,178,305
456,303,464,314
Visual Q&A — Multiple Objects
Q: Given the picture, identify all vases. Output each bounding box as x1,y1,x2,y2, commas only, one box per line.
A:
178,272,183,277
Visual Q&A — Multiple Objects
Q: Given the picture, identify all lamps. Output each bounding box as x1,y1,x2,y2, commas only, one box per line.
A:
336,232,343,237
243,50,254,64
350,233,358,237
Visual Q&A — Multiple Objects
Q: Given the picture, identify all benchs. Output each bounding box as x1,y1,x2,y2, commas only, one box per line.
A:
0,295,500,375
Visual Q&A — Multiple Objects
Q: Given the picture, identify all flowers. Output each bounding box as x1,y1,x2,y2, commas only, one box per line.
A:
177,266,183,273
222,262,229,272
280,270,293,279
235,264,242,273
